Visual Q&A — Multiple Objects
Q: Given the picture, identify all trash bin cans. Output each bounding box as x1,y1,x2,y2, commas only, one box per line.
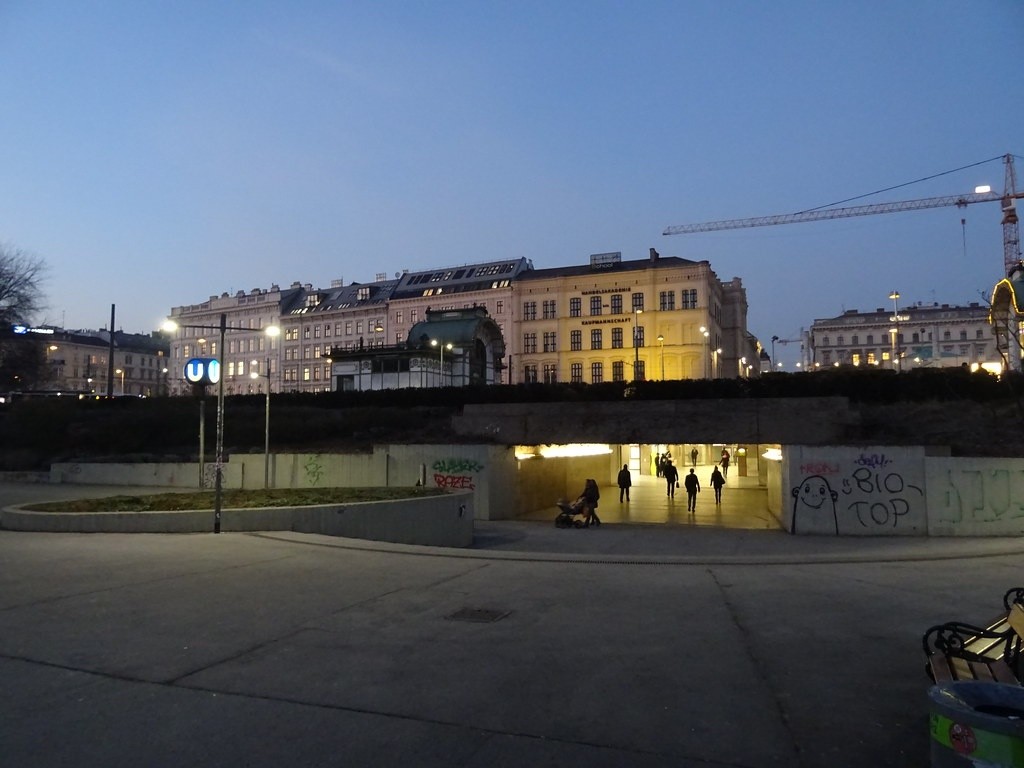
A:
919,680,1024,768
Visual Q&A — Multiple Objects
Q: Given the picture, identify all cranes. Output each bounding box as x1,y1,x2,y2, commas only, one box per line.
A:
778,325,806,371
661,152,1024,278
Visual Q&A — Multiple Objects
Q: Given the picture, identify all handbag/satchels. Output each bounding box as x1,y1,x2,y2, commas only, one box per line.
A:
676,481,679,488
720,477,725,484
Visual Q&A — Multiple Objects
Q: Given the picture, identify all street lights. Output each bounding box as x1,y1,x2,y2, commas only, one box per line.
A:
890,290,902,372
373,325,383,347
157,368,168,397
99,328,115,399
163,319,280,534
635,308,644,378
701,327,710,379
116,369,124,395
46,345,57,360
431,339,453,388
771,335,778,372
250,359,270,491
658,335,664,381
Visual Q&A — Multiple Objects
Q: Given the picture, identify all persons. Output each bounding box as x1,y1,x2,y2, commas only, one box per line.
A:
710,465,723,504
579,478,601,527
719,447,730,477
733,451,737,466
960,362,987,374
691,447,698,467
663,461,678,498
685,468,700,512
654,451,671,478
617,464,632,503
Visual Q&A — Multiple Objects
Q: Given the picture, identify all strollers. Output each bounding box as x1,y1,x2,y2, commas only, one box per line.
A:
555,494,588,529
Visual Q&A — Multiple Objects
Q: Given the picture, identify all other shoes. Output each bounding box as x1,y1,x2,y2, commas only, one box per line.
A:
692,507,695,512
627,498,629,501
620,500,623,503
688,508,690,511
